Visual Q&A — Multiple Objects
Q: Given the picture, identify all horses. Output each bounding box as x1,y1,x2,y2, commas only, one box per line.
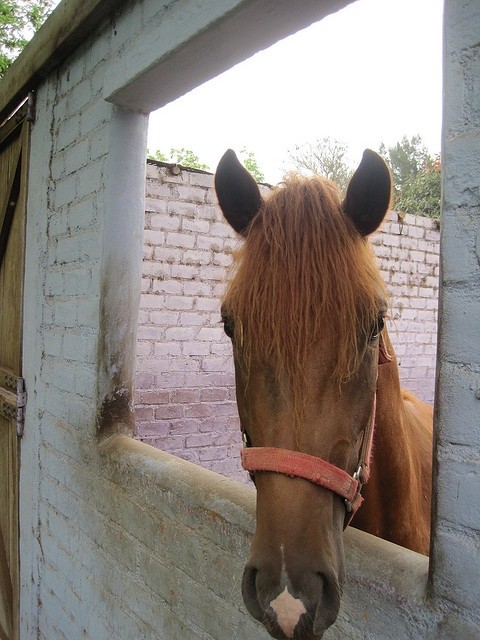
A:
213,148,434,640
